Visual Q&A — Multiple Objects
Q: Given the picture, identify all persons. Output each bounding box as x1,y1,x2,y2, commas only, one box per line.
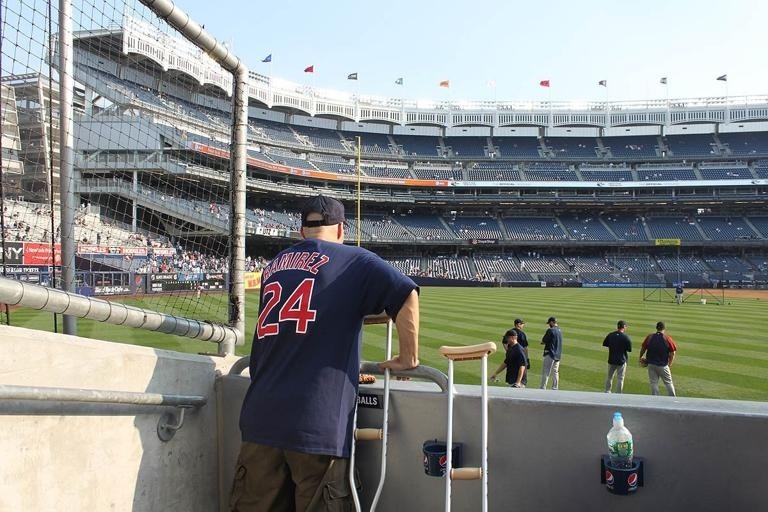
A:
196,282,201,298
538,316,562,389
502,318,531,369
230,192,421,511
640,320,677,396
491,330,528,388
603,320,632,393
676,284,683,304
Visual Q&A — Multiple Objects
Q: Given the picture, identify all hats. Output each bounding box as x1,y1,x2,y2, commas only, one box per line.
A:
301,195,346,228
546,317,557,324
515,319,524,323
503,330,518,336
656,322,665,328
617,320,627,328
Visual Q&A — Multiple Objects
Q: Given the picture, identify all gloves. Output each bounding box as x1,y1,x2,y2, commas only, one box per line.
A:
511,383,520,388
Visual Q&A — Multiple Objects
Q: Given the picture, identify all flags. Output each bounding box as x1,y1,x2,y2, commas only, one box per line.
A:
395,78,403,85
347,73,357,80
262,54,271,62
304,65,313,73
